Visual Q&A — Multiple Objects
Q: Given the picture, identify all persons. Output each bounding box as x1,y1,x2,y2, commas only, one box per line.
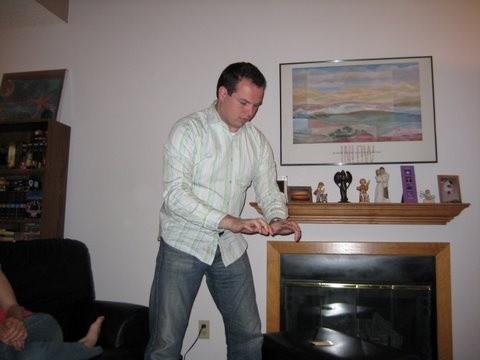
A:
143,61,302,360
314,182,328,203
375,167,390,202
0,270,104,360
356,179,370,203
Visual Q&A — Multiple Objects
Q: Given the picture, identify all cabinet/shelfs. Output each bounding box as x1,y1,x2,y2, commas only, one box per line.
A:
0,120,71,240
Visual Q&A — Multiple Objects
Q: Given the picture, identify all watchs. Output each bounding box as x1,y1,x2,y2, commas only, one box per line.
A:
269,218,283,226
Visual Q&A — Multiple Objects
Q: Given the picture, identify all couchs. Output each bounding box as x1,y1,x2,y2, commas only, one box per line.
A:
0,238,150,360
261,325,411,360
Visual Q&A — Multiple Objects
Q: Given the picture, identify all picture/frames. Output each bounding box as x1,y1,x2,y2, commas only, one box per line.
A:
438,175,461,204
279,56,438,166
0,69,69,122
287,185,312,203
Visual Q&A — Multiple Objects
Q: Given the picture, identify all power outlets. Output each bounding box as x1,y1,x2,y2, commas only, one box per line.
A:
199,320,210,339
